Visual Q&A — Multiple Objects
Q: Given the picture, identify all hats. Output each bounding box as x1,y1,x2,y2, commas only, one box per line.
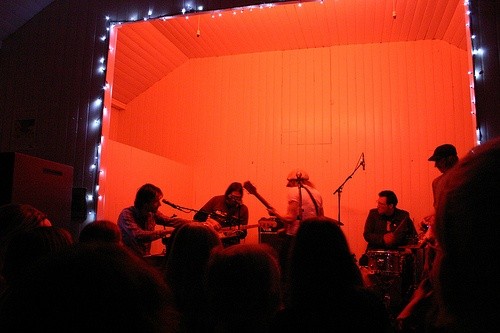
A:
427,143,457,160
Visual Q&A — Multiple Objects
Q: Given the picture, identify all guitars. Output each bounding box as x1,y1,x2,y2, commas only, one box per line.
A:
243,181,279,216
193,210,277,239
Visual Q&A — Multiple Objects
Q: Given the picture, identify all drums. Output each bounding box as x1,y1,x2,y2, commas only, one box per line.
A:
367,249,405,276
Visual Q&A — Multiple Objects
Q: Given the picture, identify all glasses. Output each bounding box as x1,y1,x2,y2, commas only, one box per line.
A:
376,200,386,206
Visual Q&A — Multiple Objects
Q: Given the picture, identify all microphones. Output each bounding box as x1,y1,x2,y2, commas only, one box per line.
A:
162,199,180,210
362,153,365,170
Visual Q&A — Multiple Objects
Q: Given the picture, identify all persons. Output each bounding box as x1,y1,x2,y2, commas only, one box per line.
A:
194,182,249,249
284,171,324,220
118,183,191,257
362,190,420,250
0,138,500,333
427,143,461,209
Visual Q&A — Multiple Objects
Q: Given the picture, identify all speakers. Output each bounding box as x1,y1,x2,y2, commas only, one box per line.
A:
0,151,74,229
